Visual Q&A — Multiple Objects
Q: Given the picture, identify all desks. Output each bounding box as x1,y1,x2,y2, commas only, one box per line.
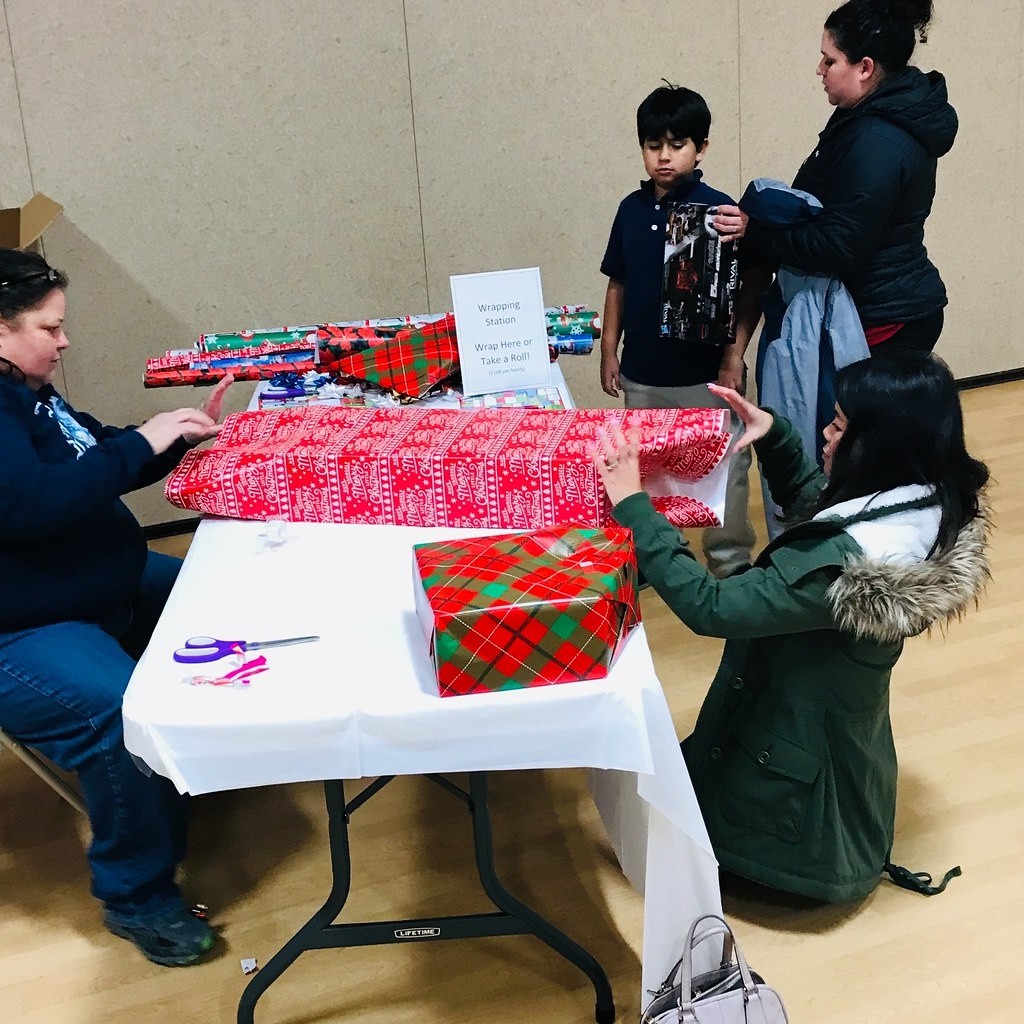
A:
119,355,725,1024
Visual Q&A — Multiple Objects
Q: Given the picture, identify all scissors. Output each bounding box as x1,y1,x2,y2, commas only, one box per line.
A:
172,636,321,664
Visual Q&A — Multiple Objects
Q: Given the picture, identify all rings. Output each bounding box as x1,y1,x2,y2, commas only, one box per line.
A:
608,462,618,470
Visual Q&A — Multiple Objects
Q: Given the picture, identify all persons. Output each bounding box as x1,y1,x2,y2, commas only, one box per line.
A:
589,351,995,903
599,77,774,591
1,247,235,966
713,0,958,475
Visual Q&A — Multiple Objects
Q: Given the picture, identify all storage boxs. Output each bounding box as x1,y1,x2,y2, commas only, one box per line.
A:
411,521,641,698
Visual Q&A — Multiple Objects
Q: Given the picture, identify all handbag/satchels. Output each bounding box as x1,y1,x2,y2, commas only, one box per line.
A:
641,913,788,1024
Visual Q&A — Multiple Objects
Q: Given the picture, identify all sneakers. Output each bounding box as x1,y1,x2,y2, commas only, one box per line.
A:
103,905,216,966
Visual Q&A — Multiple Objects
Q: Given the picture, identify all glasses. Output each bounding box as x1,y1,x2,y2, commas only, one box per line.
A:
0,263,68,288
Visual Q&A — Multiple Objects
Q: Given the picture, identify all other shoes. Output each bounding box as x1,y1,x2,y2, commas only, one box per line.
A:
622,562,649,591
